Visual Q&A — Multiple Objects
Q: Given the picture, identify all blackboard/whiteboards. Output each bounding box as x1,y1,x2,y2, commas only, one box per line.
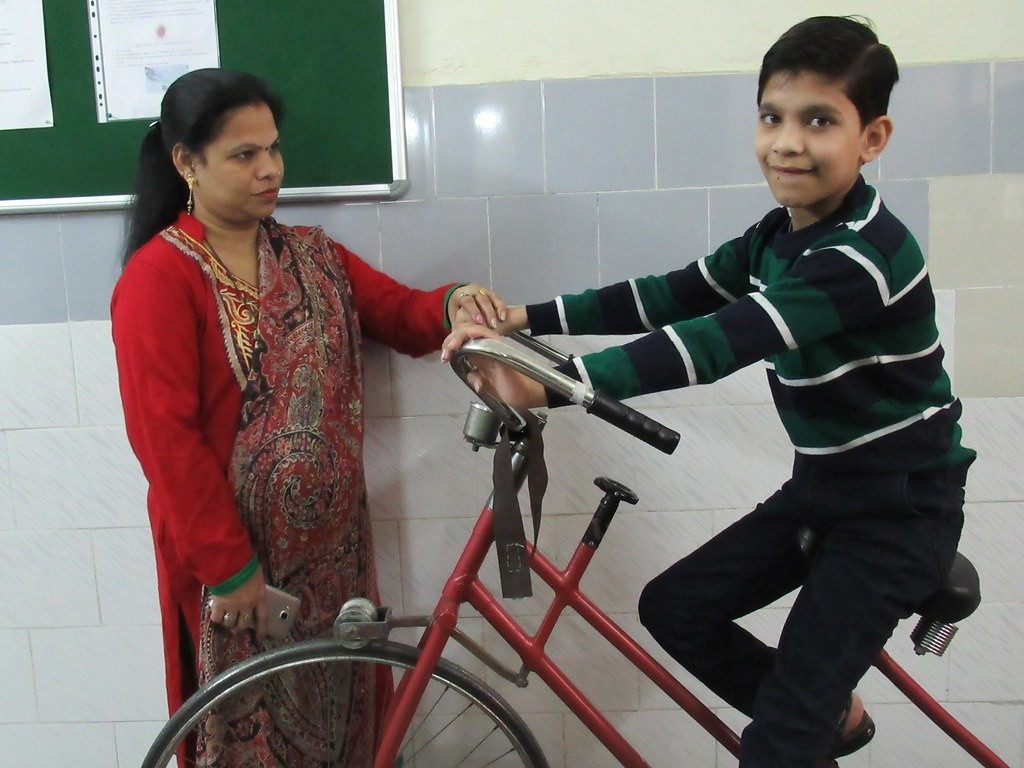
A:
0,0,415,213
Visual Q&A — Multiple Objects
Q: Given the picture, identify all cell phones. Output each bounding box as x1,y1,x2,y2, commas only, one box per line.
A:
208,584,302,640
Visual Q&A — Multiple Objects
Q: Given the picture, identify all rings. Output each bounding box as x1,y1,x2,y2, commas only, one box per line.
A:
474,288,486,299
222,610,237,623
456,292,472,299
240,610,252,623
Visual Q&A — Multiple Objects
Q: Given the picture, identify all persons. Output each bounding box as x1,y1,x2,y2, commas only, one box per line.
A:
109,69,507,768
440,15,978,768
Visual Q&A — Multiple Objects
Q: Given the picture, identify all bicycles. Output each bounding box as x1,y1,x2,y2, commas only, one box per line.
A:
141,316,1022,768
